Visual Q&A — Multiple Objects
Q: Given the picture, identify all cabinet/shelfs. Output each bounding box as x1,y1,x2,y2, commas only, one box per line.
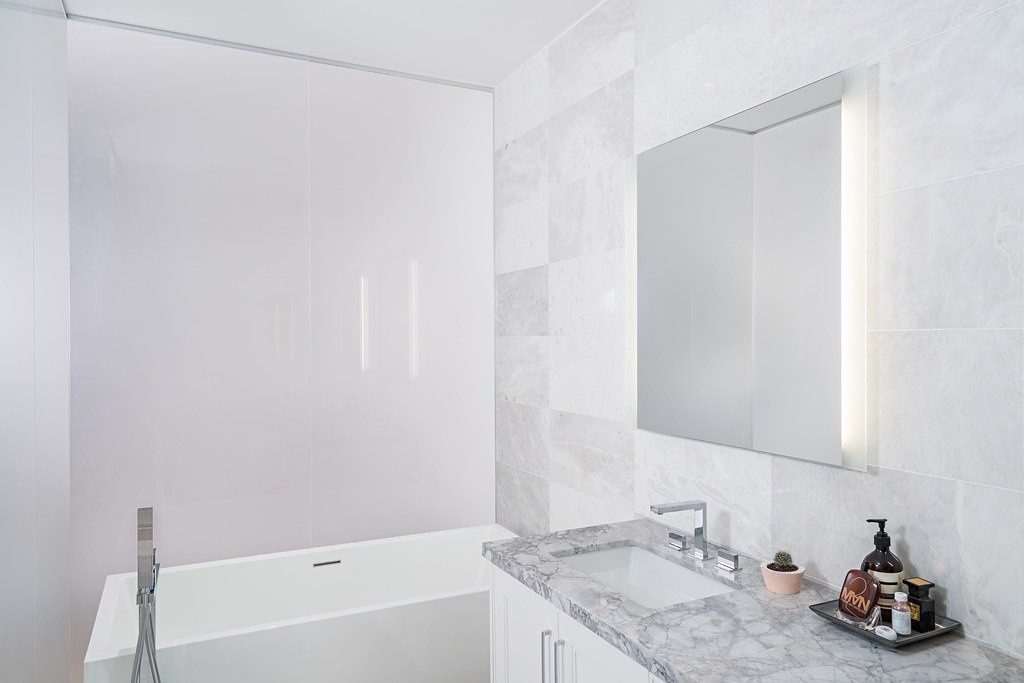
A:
489,564,665,683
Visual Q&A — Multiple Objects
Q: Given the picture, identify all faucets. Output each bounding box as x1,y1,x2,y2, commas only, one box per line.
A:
650,498,715,562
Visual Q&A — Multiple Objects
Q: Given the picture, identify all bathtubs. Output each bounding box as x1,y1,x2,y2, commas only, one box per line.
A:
82,523,518,683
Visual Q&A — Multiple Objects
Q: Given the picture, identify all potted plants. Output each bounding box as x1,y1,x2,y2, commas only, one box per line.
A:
760,551,806,594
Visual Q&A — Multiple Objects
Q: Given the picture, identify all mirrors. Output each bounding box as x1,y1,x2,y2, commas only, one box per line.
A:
636,69,871,471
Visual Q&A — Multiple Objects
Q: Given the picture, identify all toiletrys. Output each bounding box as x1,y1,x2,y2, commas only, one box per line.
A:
832,514,937,642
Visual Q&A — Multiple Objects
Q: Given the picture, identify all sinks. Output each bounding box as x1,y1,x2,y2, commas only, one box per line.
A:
548,532,747,614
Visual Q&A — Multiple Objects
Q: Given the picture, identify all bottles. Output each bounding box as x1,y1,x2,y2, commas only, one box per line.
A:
891,592,912,636
